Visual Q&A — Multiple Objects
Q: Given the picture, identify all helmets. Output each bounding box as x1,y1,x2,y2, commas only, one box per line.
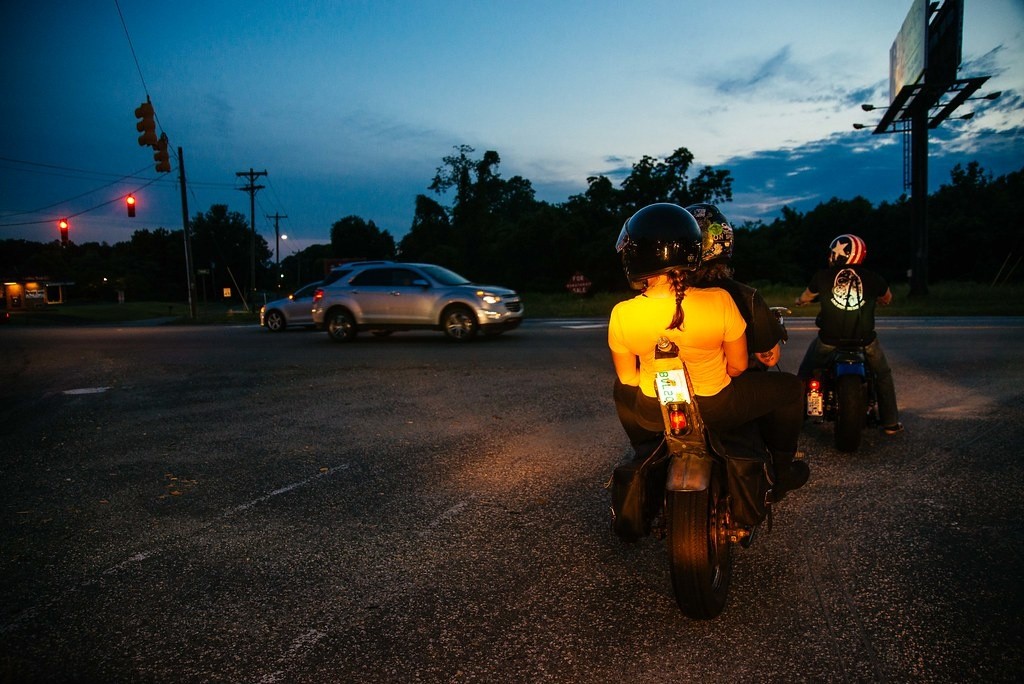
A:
615,202,702,282
829,234,867,265
685,201,734,273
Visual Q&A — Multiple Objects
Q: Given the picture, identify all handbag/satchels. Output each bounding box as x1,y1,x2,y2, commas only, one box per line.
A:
726,456,772,526
612,436,670,541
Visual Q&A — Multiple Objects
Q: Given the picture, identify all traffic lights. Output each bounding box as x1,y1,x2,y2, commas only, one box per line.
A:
135,102,157,147
59,218,68,241
127,196,135,218
152,138,171,173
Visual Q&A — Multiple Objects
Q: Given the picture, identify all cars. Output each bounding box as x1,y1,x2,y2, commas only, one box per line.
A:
260,279,320,331
325,260,395,285
311,263,525,342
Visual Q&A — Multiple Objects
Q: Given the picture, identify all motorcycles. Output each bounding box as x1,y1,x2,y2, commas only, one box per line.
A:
798,290,896,453
608,304,803,624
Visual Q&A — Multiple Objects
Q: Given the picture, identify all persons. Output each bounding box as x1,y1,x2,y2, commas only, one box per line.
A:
796,234,904,434
606,203,813,503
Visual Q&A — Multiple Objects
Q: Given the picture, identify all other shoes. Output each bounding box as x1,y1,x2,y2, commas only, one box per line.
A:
767,460,809,502
883,422,905,434
